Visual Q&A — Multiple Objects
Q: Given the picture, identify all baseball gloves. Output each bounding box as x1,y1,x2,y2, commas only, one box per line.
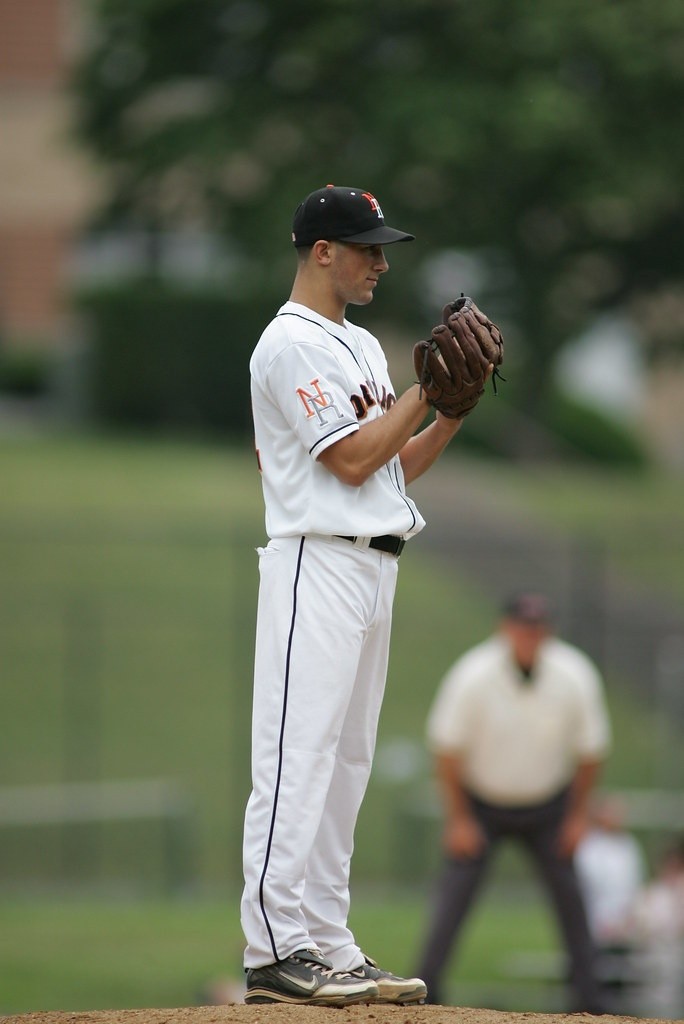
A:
408,292,510,420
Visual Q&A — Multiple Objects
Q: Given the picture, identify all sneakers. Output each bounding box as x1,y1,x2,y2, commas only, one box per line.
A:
341,952,428,1007
244,949,378,1005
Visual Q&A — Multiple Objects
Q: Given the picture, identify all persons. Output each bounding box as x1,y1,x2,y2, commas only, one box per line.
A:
422,589,684,1024
240,183,492,1006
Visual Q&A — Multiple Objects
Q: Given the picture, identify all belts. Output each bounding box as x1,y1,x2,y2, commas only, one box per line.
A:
333,532,406,562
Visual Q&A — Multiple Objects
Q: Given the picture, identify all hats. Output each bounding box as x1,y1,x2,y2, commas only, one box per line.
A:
292,184,415,245
504,590,554,627
